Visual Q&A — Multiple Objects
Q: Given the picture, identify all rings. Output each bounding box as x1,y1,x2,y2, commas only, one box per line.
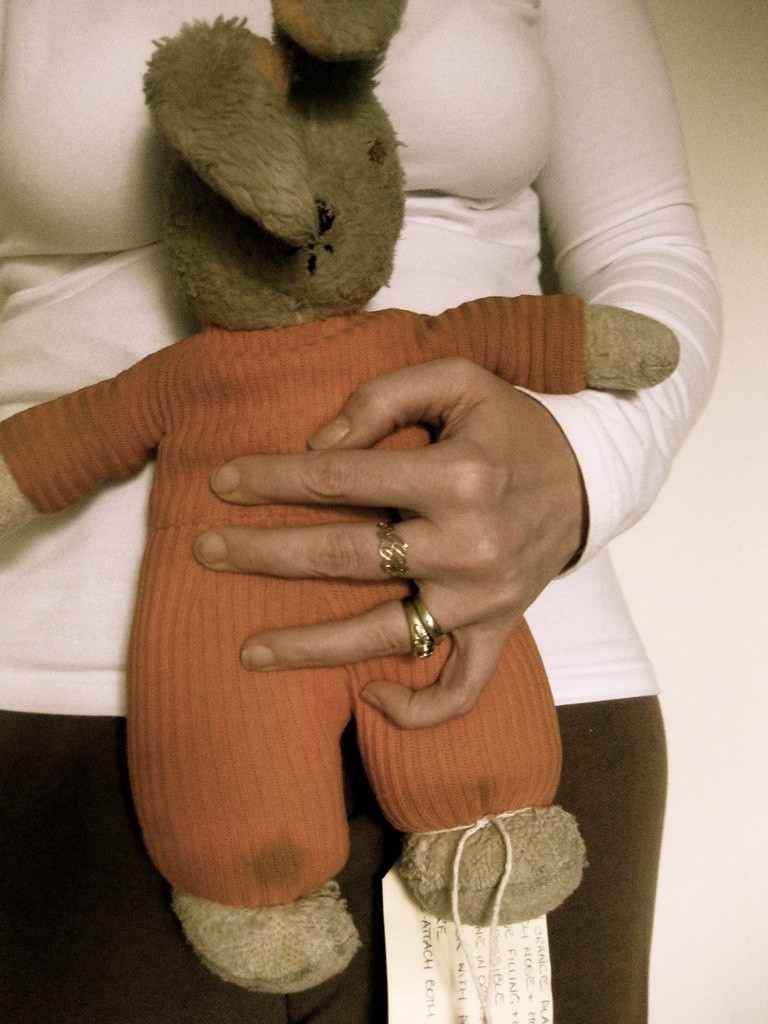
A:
378,523,410,578
401,597,434,659
412,596,446,646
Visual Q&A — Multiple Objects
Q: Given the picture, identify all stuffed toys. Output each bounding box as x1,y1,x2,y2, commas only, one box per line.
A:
0,0,682,994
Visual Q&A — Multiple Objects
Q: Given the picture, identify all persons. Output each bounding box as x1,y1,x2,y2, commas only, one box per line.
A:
0,0,723,1024
